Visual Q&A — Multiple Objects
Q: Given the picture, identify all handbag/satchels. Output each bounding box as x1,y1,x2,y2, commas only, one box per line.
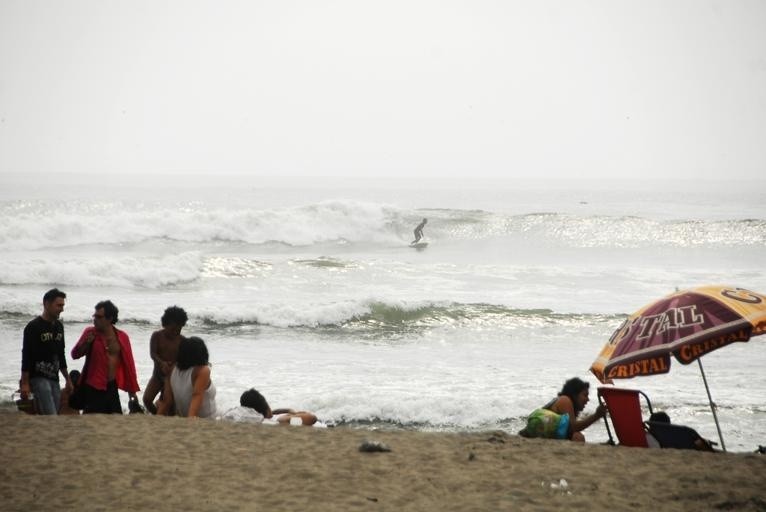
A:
69,383,89,409
16,399,37,415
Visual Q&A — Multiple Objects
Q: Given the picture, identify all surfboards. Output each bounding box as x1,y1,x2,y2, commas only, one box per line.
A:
409,242,428,249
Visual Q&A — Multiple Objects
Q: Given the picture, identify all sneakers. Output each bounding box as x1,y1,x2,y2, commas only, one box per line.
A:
129,399,146,413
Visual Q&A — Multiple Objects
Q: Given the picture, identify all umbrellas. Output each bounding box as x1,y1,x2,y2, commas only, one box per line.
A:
589,283,766,452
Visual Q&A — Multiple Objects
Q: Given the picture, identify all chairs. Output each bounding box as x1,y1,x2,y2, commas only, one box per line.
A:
644,420,715,452
597,387,654,448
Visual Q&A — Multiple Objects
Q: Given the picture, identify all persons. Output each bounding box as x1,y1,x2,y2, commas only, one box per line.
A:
542,376,607,441
647,411,671,448
413,218,428,243
20,287,73,415
58,370,80,415
142,306,188,414
68,301,140,414
161,337,264,422
240,388,328,429
